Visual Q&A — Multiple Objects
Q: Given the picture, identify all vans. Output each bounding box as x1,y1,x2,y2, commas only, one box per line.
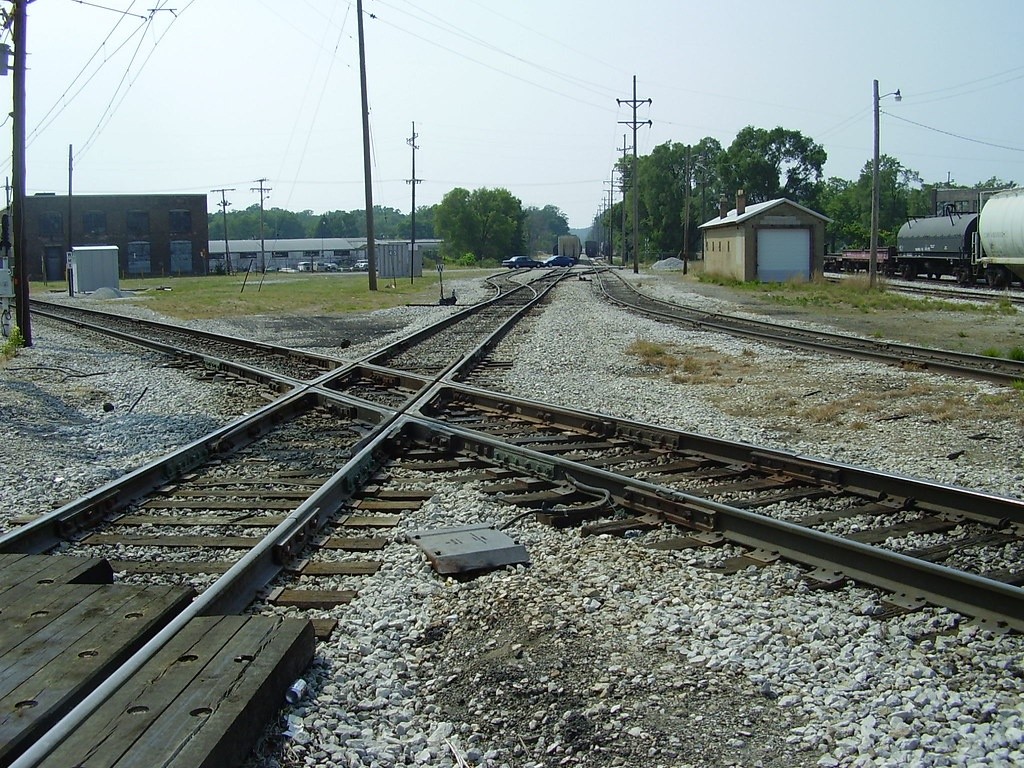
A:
298,262,311,272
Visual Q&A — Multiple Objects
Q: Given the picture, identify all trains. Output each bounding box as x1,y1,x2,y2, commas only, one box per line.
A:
585,240,598,257
558,235,582,260
823,184,1024,290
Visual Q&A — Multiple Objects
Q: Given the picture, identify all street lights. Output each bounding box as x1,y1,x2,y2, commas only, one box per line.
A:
868,90,903,287
260,196,270,273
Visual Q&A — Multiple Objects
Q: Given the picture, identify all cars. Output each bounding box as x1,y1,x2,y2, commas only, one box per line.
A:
359,263,368,271
542,255,574,267
502,256,544,269
